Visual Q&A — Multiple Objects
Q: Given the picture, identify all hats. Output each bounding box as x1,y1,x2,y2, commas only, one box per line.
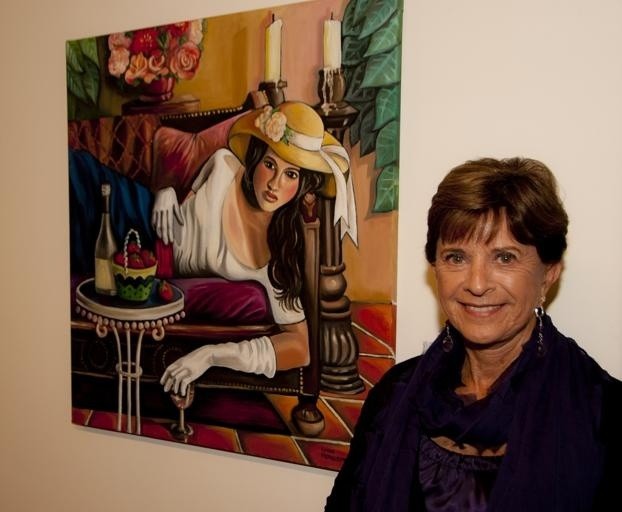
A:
229,100,348,174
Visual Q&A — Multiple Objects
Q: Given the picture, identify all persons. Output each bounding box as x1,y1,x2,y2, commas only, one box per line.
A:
322,153,621,511
69,99,353,400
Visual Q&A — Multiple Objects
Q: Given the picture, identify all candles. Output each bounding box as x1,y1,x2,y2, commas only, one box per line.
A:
263,11,283,83
321,8,345,68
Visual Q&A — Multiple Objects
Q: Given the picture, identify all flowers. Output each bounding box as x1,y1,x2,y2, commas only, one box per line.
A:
104,20,205,84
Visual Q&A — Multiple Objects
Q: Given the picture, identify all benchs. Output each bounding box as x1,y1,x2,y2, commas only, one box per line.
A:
67,91,325,437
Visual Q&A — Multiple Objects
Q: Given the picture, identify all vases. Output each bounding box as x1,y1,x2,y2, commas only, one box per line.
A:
133,74,178,102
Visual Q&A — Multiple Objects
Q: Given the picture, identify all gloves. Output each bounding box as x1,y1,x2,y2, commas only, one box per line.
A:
160,334,277,396
153,186,183,243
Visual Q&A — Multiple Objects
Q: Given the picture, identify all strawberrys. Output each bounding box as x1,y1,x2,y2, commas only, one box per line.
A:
156,280,173,302
113,243,156,269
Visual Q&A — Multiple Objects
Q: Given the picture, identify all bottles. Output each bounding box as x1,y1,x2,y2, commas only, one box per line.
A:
94,184,121,298
